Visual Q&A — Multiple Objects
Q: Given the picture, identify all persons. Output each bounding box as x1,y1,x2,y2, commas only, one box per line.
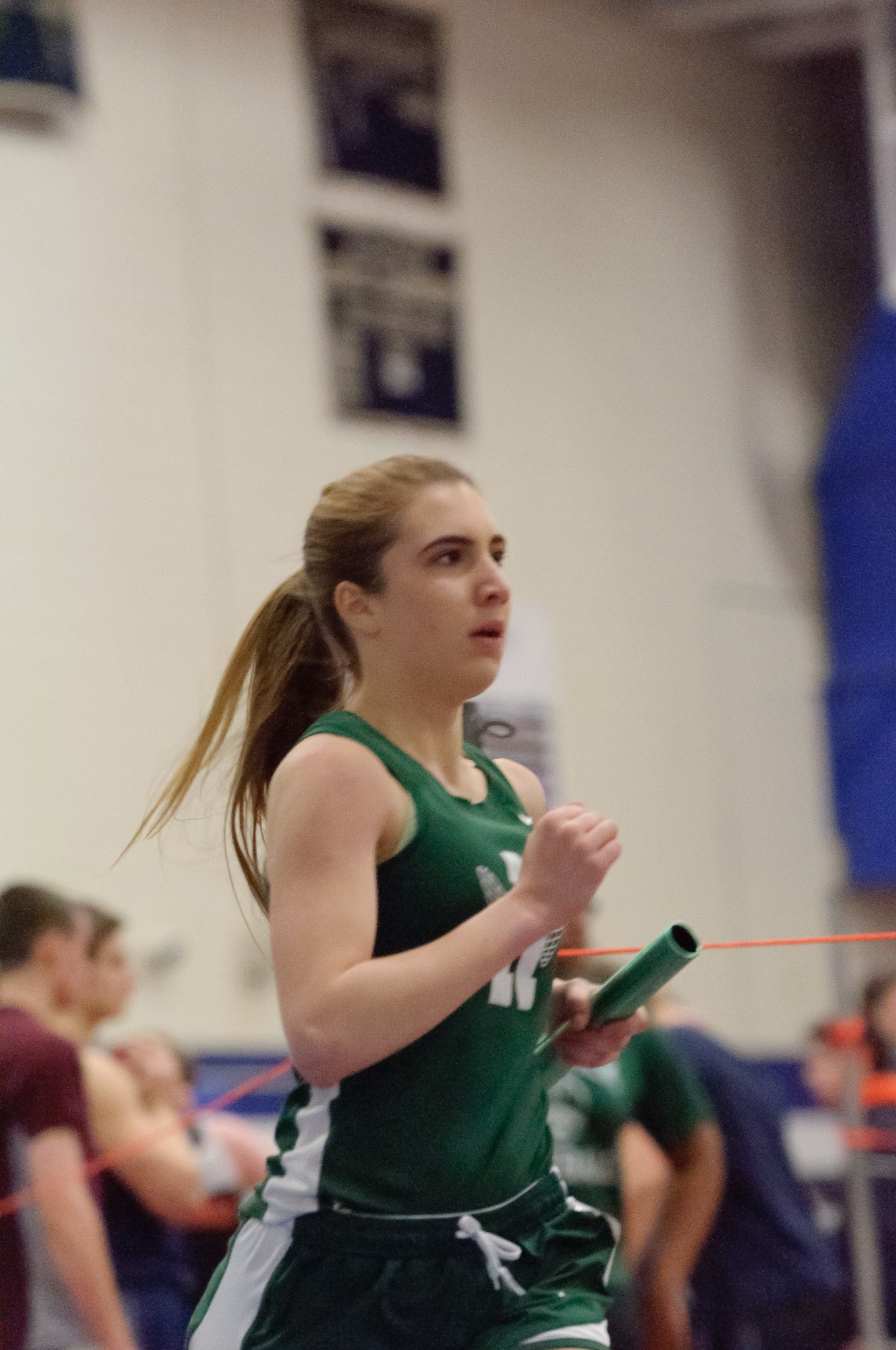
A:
0,884,273,1350
102,450,652,1350
797,973,896,1163
583,963,858,1349
